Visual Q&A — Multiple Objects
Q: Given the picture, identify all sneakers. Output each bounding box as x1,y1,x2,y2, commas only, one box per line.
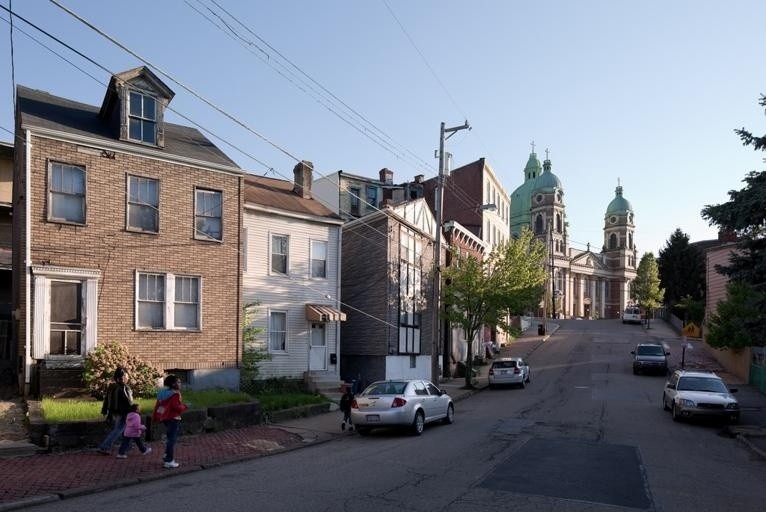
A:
96,445,179,467
342,423,345,430
349,427,353,431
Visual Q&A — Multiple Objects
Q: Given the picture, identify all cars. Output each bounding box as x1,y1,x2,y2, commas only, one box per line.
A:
486,354,532,390
630,339,672,377
662,366,742,424
347,370,456,437
622,304,643,325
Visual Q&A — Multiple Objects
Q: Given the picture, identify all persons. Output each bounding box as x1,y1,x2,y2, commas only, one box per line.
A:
338,386,355,433
96,366,134,456
116,403,152,459
151,375,192,469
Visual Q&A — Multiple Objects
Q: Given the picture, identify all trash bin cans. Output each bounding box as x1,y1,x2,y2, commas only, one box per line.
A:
538,323,545,335
751,347,766,393
